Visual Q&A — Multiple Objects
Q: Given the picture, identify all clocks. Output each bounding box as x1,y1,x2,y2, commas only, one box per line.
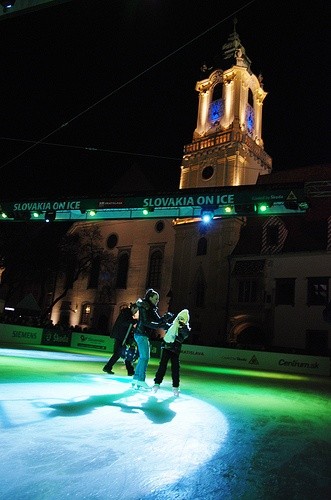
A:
246,103,256,129
207,98,225,120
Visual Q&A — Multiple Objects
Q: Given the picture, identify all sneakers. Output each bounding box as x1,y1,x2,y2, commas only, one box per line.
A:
173,387,180,396
128,371,135,376
132,380,138,387
152,384,160,392
137,381,152,391
102,367,114,375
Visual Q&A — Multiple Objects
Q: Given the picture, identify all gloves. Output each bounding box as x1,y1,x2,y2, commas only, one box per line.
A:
164,323,173,330
164,312,174,320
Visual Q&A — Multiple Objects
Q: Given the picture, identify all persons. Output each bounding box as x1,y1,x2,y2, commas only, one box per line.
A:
133,289,174,390
103,302,139,376
152,309,191,396
